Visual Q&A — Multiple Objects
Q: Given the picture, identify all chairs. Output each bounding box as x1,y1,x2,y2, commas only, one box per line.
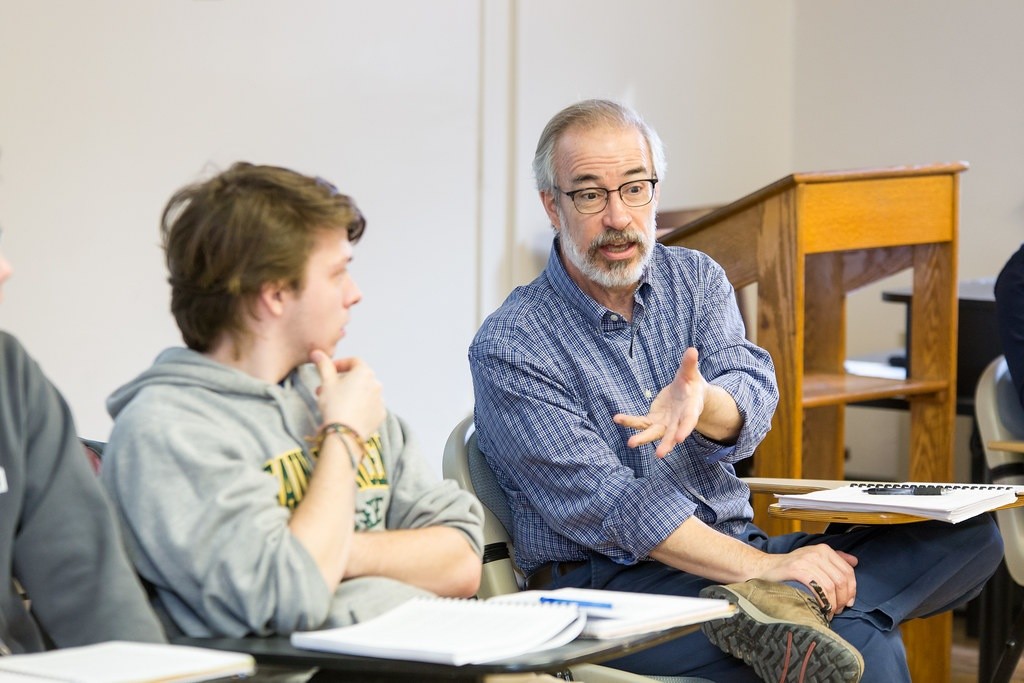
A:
974,354,1024,683
441,414,720,683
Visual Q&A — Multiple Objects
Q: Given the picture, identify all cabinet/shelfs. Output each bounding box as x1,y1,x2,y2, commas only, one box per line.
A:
657,159,969,683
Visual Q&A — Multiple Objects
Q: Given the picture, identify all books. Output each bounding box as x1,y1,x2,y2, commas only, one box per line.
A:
291,585,740,666
772,481,1019,525
0,641,257,683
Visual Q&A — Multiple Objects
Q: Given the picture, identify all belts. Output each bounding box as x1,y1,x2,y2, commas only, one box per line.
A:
523,559,590,590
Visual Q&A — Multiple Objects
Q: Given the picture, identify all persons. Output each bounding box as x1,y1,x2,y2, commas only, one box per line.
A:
97,157,583,683
468,101,1005,683
0,222,168,657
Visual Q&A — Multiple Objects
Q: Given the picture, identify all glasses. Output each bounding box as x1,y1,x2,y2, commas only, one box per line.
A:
553,168,659,216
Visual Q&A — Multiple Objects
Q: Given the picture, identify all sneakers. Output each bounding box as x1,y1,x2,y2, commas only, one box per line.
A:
697,578,866,682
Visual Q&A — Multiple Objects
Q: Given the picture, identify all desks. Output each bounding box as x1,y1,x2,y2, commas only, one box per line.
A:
144,585,737,672
737,477,1024,524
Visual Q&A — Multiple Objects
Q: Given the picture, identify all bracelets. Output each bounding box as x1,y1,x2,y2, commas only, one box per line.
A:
305,422,374,469
